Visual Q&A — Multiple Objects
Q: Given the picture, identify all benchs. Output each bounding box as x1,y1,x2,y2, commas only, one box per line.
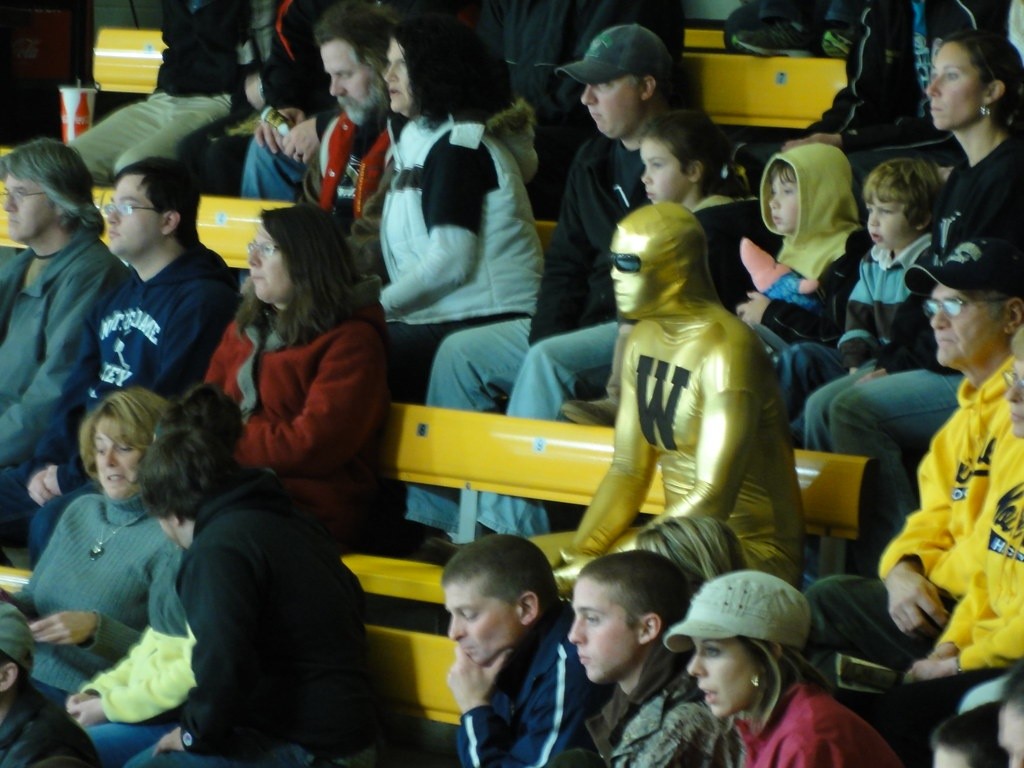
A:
1,28,880,727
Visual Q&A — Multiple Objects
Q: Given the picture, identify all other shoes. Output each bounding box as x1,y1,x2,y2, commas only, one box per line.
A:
562,397,618,428
730,19,819,57
420,536,460,566
822,28,857,58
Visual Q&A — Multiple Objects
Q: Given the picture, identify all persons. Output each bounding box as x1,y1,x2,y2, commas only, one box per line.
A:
0,602,102,768
568,550,744,768
906,324,1024,681
0,0,1024,768
662,571,904,768
441,535,612,768
124,429,386,768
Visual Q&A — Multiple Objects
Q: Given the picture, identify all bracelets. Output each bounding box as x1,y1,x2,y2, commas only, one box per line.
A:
955,654,962,675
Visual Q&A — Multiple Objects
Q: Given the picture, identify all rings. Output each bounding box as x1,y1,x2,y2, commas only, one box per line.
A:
294,152,303,157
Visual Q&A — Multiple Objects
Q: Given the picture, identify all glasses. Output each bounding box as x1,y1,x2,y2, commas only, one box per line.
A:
103,203,164,218
246,241,282,258
923,296,1001,317
606,249,642,274
1,190,46,204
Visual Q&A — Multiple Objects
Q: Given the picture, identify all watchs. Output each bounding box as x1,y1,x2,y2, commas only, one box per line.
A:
180,726,198,753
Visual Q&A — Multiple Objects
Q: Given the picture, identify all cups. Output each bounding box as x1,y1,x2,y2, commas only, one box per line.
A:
59,87,96,144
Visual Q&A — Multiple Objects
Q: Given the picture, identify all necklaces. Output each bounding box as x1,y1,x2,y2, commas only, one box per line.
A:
88,515,136,560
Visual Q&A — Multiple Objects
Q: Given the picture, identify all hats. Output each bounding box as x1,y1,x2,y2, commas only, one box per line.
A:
905,234,1024,300
554,23,673,85
663,570,811,651
1,601,35,674
